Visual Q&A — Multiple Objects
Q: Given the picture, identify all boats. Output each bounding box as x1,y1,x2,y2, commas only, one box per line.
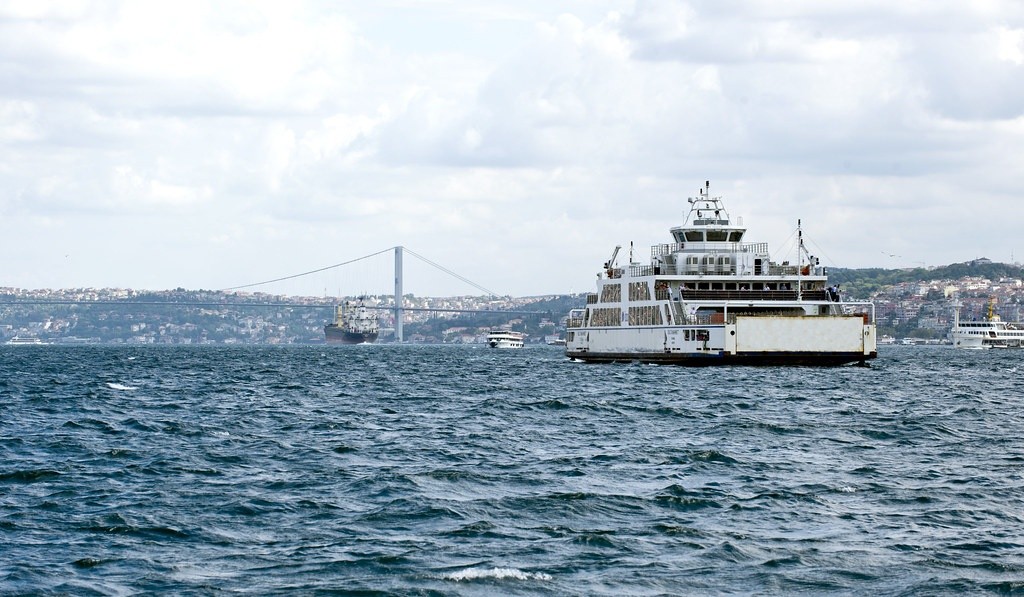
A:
947,322,1024,350
549,339,566,345
486,331,524,348
565,178,879,364
880,334,951,345
323,298,382,343
